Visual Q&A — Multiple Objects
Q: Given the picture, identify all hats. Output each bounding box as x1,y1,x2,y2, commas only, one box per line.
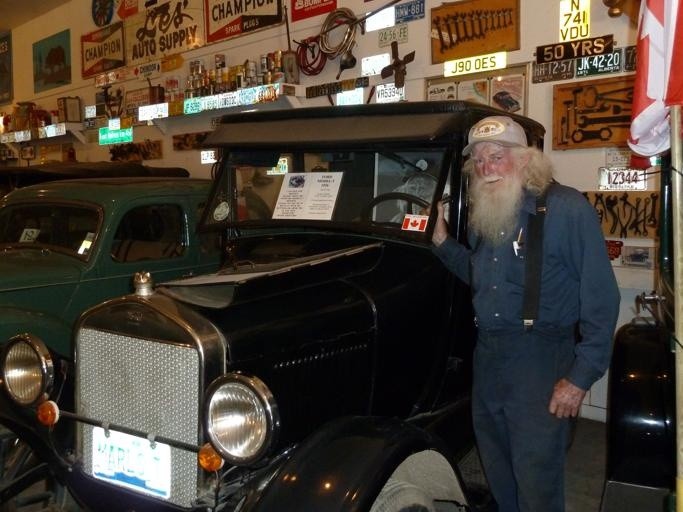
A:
461,115,528,154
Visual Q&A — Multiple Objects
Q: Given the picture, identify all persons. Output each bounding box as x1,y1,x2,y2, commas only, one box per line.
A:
416,114,621,512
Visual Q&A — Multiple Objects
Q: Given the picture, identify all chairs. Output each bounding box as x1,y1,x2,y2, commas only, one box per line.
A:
116,209,179,259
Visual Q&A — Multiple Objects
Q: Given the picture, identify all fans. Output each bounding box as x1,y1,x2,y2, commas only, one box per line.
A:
381,41,415,88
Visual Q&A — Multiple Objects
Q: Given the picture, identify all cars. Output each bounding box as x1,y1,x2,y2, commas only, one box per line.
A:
601,151,676,512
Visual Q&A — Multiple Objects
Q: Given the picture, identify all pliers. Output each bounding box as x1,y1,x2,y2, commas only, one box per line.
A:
594,193,651,239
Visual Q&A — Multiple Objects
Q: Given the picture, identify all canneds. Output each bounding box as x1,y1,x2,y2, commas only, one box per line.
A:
184,50,287,98
